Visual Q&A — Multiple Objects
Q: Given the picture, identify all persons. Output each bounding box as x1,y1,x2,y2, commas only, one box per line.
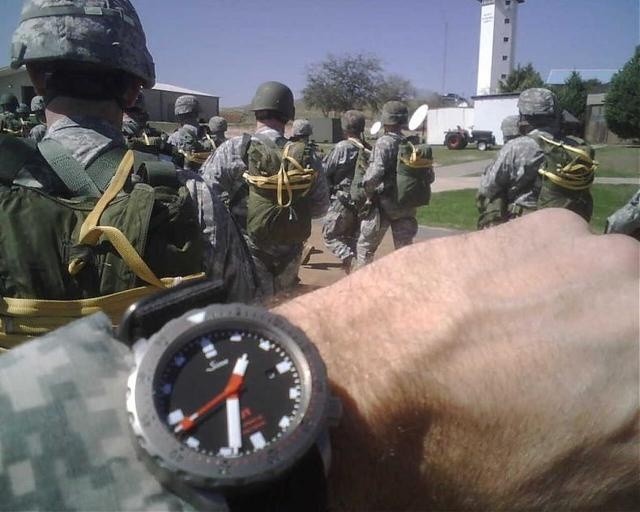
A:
0,206,640,512
0,79,330,298
320,100,419,273
482,115,522,174
0,0,266,356
476,86,594,231
603,190,640,240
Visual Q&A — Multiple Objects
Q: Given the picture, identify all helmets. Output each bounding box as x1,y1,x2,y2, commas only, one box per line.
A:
517,88,561,116
503,116,522,138
10,0,155,91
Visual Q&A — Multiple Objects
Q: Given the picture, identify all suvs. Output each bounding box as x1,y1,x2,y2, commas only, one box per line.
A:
440,93,465,105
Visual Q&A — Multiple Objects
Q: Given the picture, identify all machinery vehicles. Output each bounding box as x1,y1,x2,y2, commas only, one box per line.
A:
443,125,495,151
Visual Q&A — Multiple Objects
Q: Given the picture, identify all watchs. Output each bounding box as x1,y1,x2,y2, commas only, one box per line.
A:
120,275,343,511
